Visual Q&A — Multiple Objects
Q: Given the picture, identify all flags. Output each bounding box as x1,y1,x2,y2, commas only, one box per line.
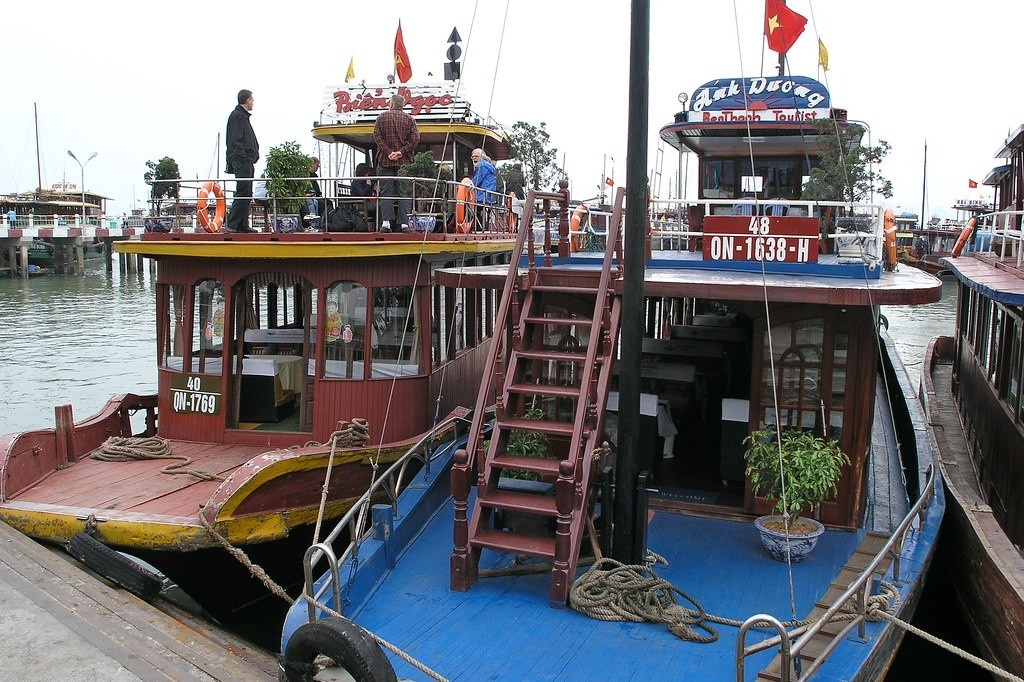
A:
969,179,978,188
818,37,829,71
393,18,412,84
345,56,355,82
763,0,808,55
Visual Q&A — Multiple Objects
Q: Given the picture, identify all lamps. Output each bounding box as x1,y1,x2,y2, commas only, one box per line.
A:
387,74,393,84
677,92,688,112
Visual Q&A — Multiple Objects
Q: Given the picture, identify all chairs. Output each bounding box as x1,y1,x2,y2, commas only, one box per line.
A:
733,197,759,216
763,198,790,216
336,183,376,232
819,206,832,254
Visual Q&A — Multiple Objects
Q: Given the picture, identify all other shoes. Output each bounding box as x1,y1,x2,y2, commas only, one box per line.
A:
303,213,321,221
227,226,258,233
402,227,410,233
379,227,392,233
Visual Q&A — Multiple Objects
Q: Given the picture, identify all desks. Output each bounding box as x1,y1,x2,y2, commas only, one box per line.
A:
240,354,303,422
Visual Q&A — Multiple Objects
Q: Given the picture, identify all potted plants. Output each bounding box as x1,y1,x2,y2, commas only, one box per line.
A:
397,150,436,233
143,156,181,233
744,425,852,562
263,140,312,233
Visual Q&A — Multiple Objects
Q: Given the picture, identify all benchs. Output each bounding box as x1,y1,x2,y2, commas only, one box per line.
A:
166,307,437,423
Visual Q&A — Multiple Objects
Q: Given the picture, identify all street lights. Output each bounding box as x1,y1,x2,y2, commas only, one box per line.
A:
67,150,97,235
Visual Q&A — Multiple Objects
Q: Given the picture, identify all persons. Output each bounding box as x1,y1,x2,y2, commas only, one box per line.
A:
7,209,16,229
374,95,420,231
224,88,259,233
471,148,497,231
253,156,382,231
505,163,527,200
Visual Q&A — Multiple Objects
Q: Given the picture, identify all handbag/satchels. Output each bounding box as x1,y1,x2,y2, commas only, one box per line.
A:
327,207,368,232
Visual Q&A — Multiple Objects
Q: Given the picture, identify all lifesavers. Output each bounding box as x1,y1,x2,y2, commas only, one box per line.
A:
198,182,225,233
951,217,976,259
883,208,897,272
282,617,395,682
456,177,475,234
570,205,588,252
506,192,518,232
66,531,165,604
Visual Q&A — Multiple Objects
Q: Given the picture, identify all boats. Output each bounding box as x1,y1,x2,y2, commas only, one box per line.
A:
0,84,562,642
885,198,998,274
915,124,1024,682
285,55,948,682
0,191,114,268
18,267,50,276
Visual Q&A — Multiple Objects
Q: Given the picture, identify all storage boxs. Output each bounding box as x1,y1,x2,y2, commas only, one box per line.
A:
703,188,719,199
